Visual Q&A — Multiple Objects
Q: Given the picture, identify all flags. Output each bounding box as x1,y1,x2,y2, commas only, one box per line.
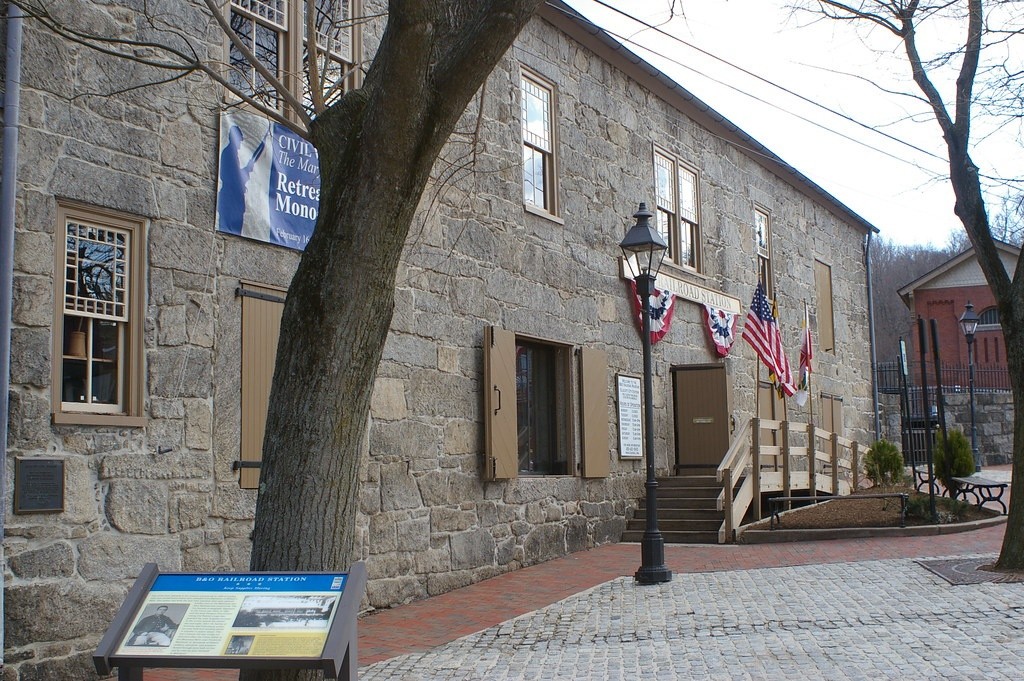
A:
796,302,812,407
741,280,798,401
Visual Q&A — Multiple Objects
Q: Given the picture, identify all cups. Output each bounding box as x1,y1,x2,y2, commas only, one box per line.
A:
68,331,86,358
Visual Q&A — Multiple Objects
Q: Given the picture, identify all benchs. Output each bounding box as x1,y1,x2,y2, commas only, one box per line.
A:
915,466,1008,515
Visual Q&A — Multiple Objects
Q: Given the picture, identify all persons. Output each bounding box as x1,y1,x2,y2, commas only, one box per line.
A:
129,606,180,646
